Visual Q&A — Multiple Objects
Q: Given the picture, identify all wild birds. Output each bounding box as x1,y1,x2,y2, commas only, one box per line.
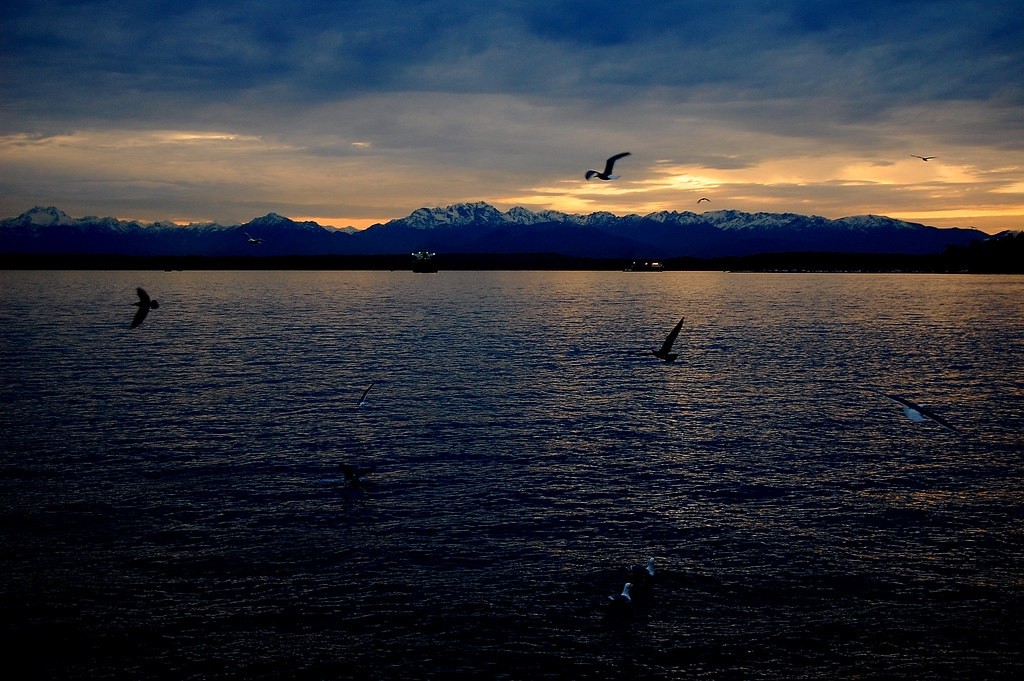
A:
697,198,711,204
129,287,159,330
910,153,937,162
600,557,656,608
853,383,963,437
585,151,632,182
651,316,685,365
356,382,376,405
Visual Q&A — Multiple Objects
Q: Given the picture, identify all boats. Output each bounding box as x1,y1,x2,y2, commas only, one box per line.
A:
411,249,440,273
